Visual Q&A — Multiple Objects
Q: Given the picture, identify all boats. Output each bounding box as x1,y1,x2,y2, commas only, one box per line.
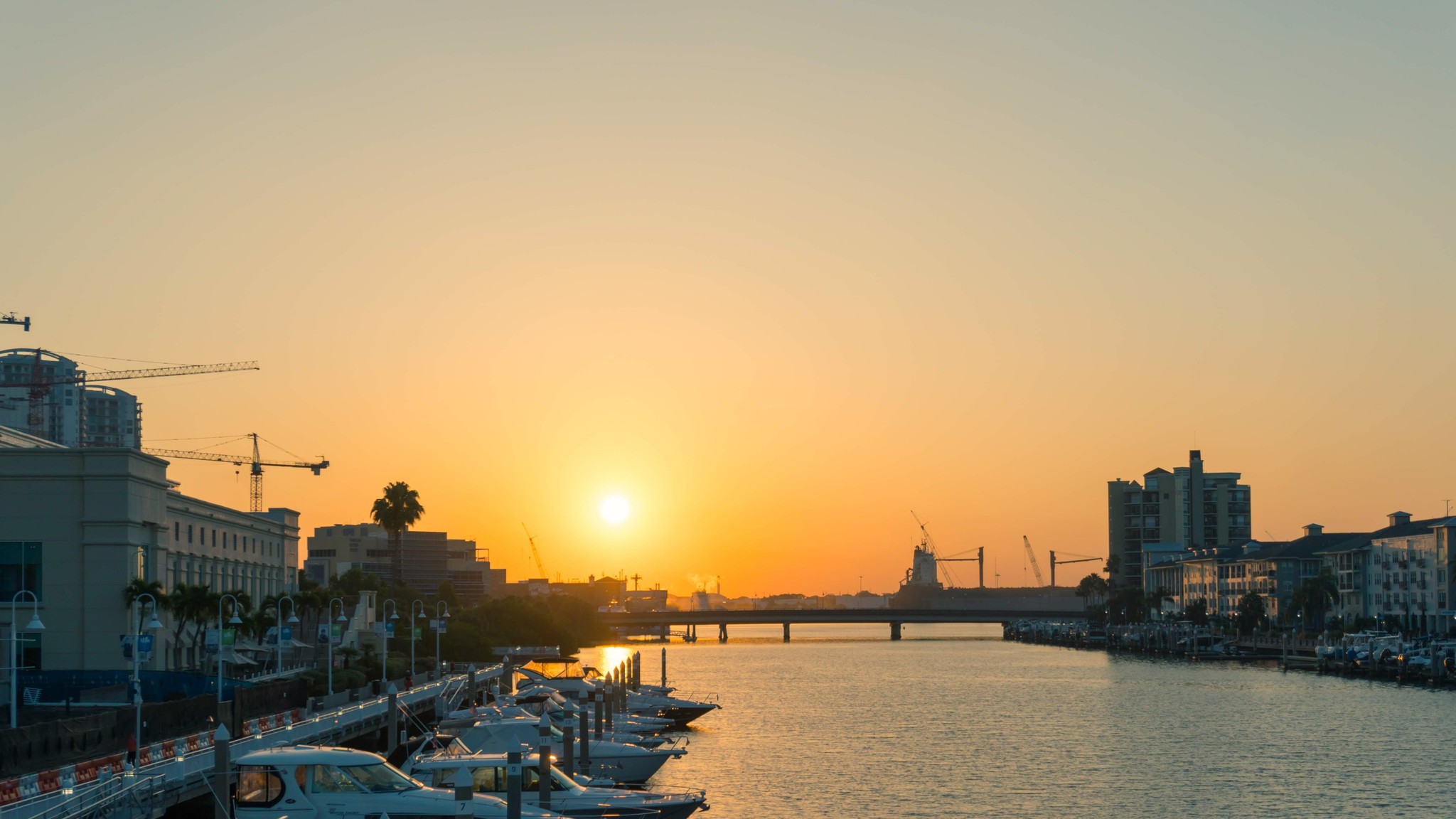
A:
1315,628,1456,679
1002,614,1254,655
360,639,722,818
231,744,663,819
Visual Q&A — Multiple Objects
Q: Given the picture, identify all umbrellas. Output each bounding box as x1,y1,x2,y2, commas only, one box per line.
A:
200,638,315,676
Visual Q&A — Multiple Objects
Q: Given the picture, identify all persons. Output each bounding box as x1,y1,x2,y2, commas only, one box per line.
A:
123,734,137,768
405,671,413,692
319,629,328,644
449,659,455,677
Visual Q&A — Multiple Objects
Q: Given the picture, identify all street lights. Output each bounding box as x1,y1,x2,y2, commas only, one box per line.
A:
1105,605,1111,625
411,599,427,687
1140,611,1146,626
1264,610,1271,632
437,601,451,670
858,576,863,608
216,594,242,703
381,599,401,681
10,590,45,728
135,593,164,779
1297,607,1304,633
1121,606,1127,625
1338,606,1346,633
1235,610,1242,629
1374,611,1378,637
328,598,348,695
277,596,300,678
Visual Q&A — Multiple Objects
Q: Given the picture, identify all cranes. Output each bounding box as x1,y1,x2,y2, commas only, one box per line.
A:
0,348,260,439
48,433,329,513
1022,532,1049,588
0,311,31,331
522,523,546,579
910,510,964,588
